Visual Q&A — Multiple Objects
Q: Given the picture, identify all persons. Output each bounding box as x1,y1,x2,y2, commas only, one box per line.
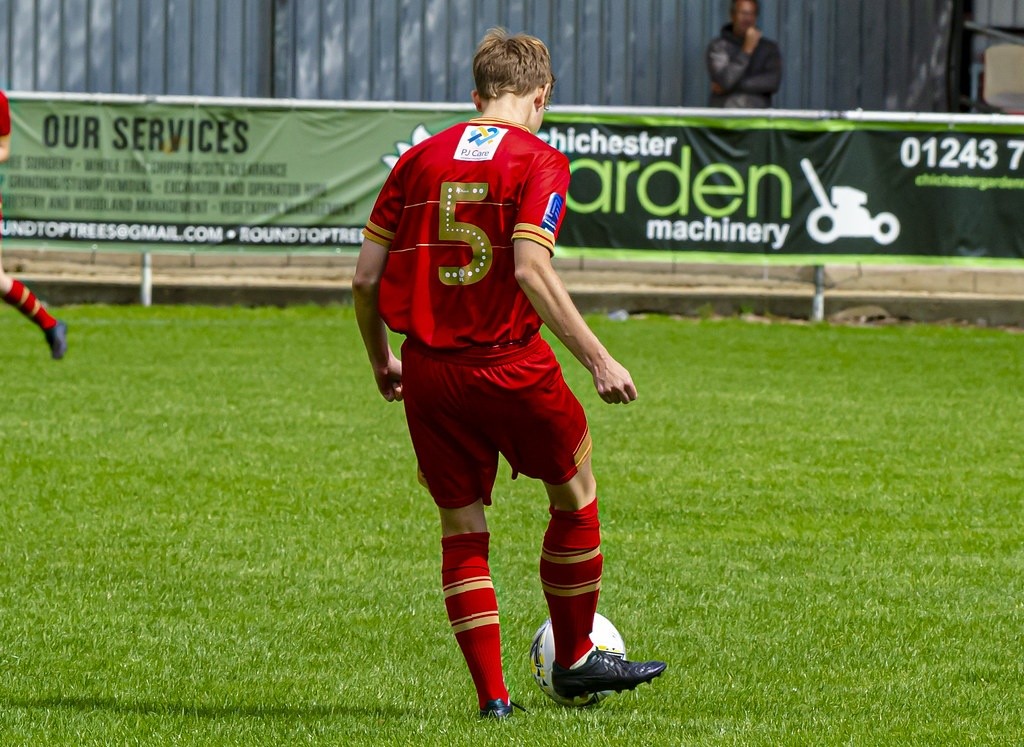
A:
0,88,66,362
351,25,669,717
705,0,784,109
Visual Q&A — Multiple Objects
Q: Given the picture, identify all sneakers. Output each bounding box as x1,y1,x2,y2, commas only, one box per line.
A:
46,319,68,360
478,697,513,719
551,647,668,699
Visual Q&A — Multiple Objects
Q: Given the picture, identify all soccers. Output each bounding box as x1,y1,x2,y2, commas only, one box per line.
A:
529,612,626,707
